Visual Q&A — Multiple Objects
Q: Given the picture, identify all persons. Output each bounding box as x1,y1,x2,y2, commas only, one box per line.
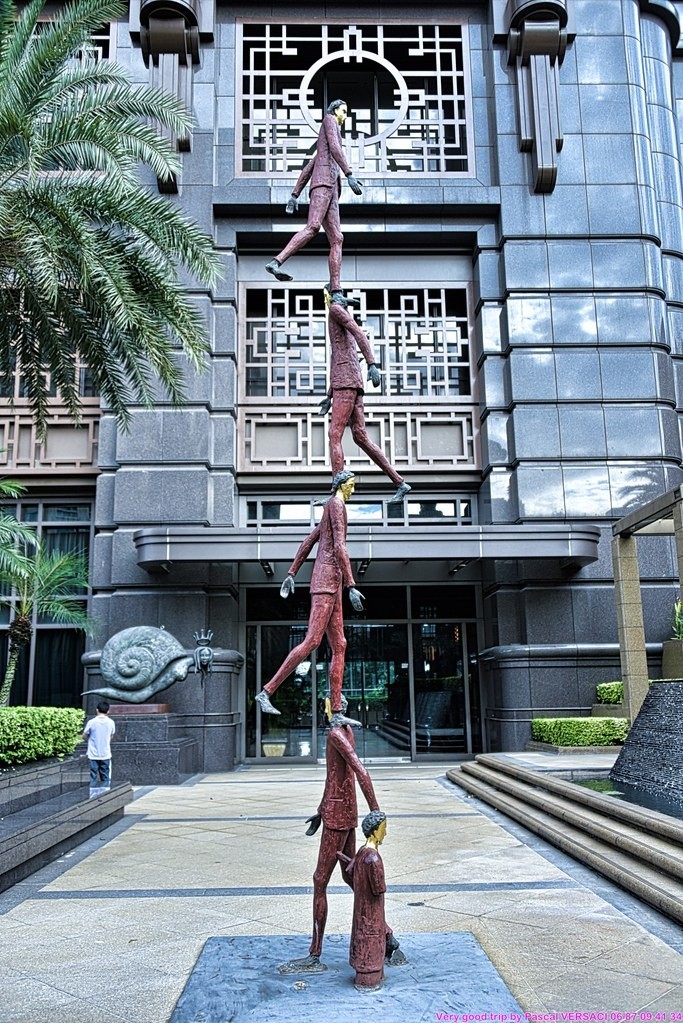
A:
82,701,115,787
90,788,108,798
255,102,411,980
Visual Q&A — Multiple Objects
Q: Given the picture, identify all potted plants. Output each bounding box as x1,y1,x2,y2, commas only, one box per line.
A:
526,718,631,755
587,679,654,718
261,706,291,757
662,600,683,677
